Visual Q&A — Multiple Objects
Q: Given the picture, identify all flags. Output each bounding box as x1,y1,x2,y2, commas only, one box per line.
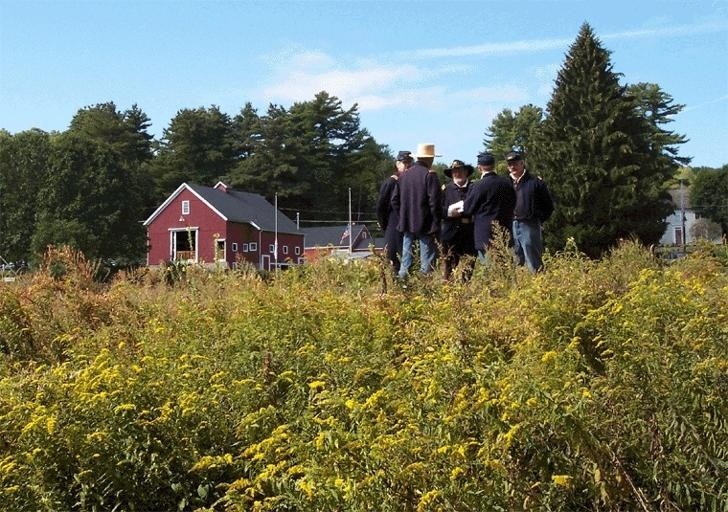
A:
339,222,351,246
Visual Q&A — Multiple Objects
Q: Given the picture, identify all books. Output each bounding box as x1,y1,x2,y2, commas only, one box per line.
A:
447,198,465,218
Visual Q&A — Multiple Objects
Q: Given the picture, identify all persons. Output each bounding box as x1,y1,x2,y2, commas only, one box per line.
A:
463,153,518,264
390,144,443,279
504,151,556,275
435,159,478,282
376,151,414,277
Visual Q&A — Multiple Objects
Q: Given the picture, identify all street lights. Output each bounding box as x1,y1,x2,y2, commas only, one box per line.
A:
678,179,692,254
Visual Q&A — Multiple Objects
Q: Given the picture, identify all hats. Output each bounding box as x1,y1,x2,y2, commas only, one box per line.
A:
476,153,496,166
396,150,414,162
407,142,443,158
442,159,475,179
504,151,522,164
